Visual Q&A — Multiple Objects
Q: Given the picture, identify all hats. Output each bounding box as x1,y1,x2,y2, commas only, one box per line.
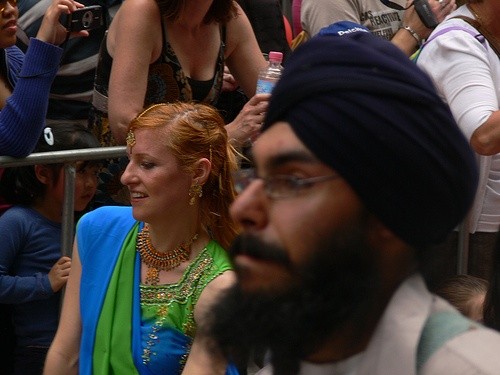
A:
259,20,478,250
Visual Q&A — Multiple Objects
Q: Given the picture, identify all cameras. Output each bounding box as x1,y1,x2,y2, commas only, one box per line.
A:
67,5,103,32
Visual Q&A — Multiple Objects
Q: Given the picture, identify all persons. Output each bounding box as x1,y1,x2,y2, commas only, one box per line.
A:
0,0,500,374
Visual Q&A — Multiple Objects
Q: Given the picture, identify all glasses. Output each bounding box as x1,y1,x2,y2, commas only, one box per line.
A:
0,0,18,13
231,166,337,202
